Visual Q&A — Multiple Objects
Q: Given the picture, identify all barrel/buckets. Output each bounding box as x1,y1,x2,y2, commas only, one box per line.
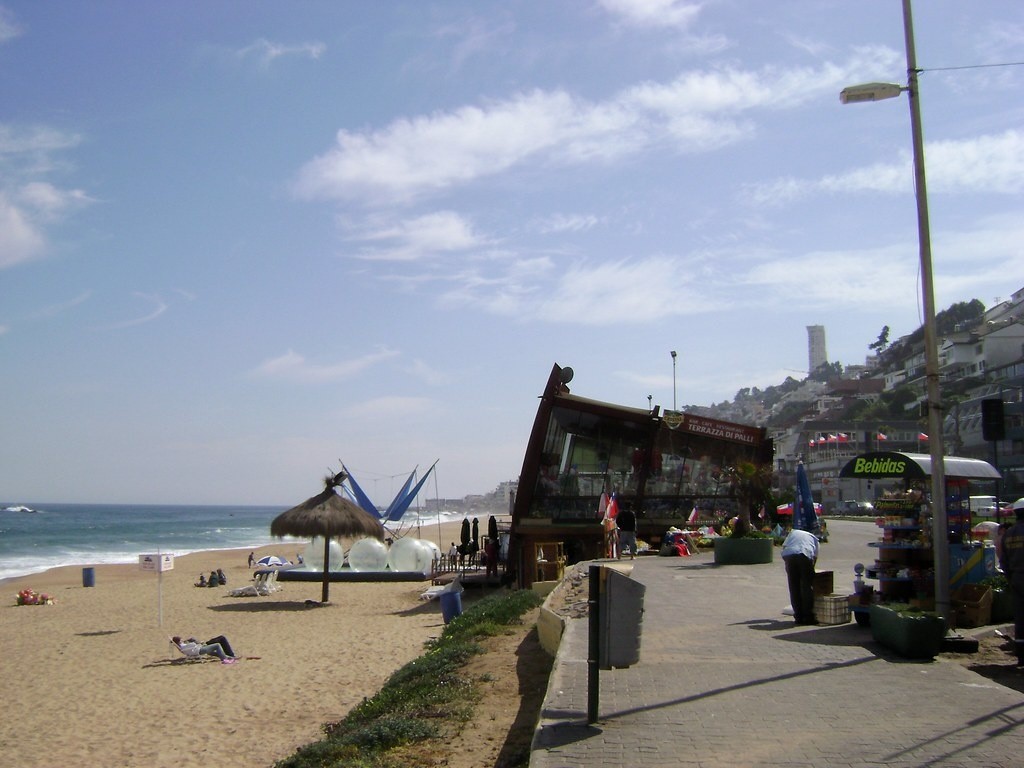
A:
83,568,95,587
440,592,462,624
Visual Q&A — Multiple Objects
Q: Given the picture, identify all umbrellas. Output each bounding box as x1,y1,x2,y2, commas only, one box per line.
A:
792,461,821,532
257,556,288,567
269,476,386,602
457,518,471,578
469,517,481,567
487,515,499,539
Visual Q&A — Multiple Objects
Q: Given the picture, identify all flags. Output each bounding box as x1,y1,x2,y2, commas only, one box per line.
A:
878,433,887,440
919,433,928,440
598,489,610,515
814,503,823,513
776,503,794,514
601,497,619,526
687,506,698,524
809,433,848,447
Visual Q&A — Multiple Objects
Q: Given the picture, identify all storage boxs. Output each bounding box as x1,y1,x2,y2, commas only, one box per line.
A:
813,593,852,625
958,605,991,627
951,582,993,607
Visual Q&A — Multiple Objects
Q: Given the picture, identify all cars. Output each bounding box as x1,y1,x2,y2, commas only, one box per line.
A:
976,501,1014,517
829,501,874,517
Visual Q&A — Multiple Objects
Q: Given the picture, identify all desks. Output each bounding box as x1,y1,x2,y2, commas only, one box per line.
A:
672,530,705,555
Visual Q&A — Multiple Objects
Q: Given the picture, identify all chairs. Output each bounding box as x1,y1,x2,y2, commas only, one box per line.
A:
266,572,274,593
224,574,260,597
257,573,270,596
273,569,283,592
534,541,569,581
167,634,209,665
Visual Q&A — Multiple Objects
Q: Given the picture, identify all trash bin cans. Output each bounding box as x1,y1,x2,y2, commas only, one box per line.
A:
594,566,647,672
82,568,96,588
438,590,463,625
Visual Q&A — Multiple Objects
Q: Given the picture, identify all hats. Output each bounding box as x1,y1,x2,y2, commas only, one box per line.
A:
1013,498,1024,510
489,539,495,545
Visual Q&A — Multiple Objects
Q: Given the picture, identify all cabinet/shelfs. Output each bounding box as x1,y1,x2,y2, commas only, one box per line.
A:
866,488,966,601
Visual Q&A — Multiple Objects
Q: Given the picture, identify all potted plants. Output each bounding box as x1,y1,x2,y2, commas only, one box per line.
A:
871,602,947,661
519,511,552,526
979,574,1012,624
713,464,774,564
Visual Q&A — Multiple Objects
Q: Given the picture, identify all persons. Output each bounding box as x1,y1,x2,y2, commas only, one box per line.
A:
195,568,226,588
448,542,457,554
172,635,243,665
1001,500,1024,667
296,553,303,565
485,539,500,577
974,520,1012,567
248,552,257,568
781,528,820,624
616,500,638,560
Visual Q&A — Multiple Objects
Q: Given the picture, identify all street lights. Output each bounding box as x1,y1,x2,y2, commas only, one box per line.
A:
838,82,953,616
647,395,652,410
669,351,677,411
